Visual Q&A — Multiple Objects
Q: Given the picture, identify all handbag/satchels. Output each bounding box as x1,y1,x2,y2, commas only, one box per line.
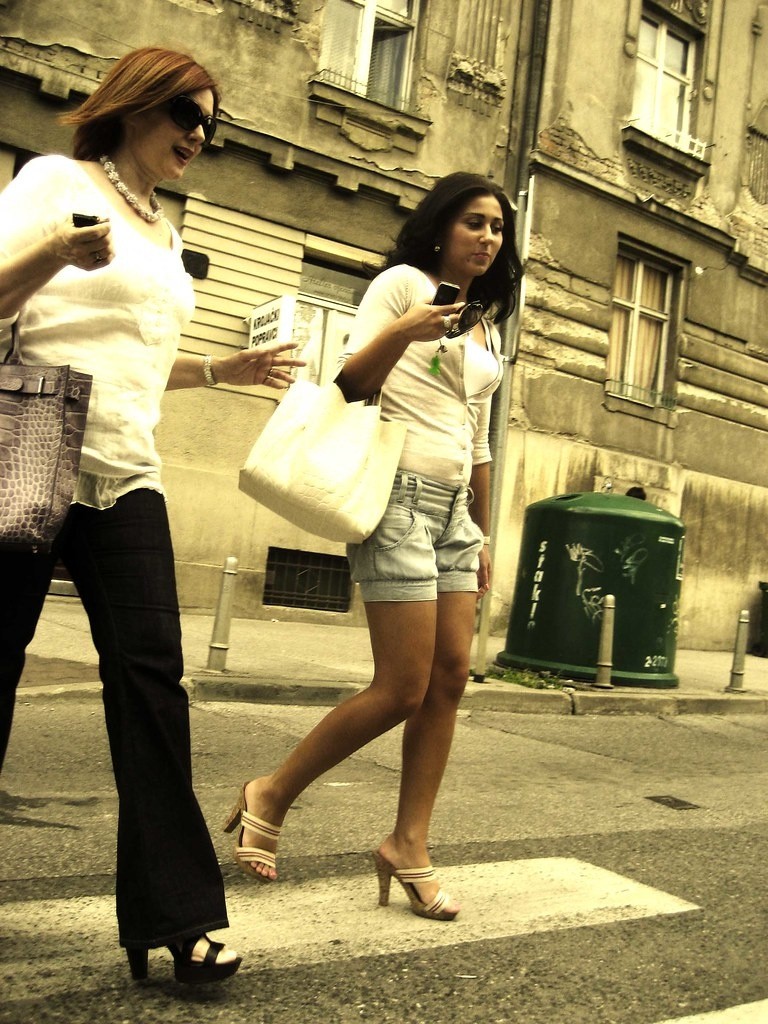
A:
0,318,93,554
238,361,408,543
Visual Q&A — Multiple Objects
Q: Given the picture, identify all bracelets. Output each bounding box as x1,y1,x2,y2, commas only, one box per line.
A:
201,355,218,386
483,536,491,546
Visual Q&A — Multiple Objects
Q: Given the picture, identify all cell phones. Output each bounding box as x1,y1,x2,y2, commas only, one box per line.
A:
72,213,99,228
432,282,461,305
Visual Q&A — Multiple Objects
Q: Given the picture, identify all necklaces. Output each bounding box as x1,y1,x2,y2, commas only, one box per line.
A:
98,153,164,223
94,251,103,261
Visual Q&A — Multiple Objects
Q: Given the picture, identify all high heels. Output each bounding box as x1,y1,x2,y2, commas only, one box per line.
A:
372,848,457,921
222,781,282,884
127,930,243,984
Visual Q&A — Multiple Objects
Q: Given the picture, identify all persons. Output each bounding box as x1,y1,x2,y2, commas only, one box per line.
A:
221,171,527,923
0,48,306,982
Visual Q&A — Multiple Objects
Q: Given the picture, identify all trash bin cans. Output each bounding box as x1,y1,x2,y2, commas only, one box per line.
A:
496,492,687,690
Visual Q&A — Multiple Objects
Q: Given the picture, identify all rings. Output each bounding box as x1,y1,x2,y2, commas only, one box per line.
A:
269,368,274,377
442,315,453,330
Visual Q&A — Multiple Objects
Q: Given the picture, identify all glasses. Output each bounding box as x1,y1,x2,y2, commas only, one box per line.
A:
165,96,218,149
445,300,483,339
